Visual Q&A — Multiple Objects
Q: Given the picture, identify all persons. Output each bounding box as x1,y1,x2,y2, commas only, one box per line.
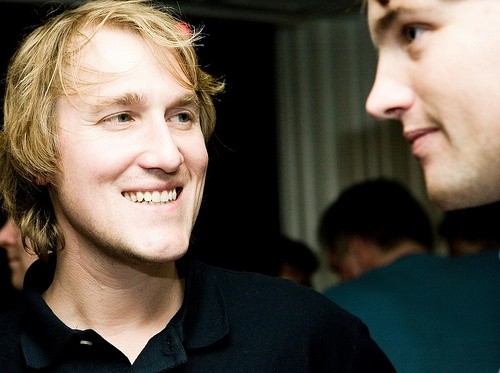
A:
236,229,319,288
0,0,400,373
366,0,500,211
0,215,40,291
316,177,500,373
438,200,500,255
